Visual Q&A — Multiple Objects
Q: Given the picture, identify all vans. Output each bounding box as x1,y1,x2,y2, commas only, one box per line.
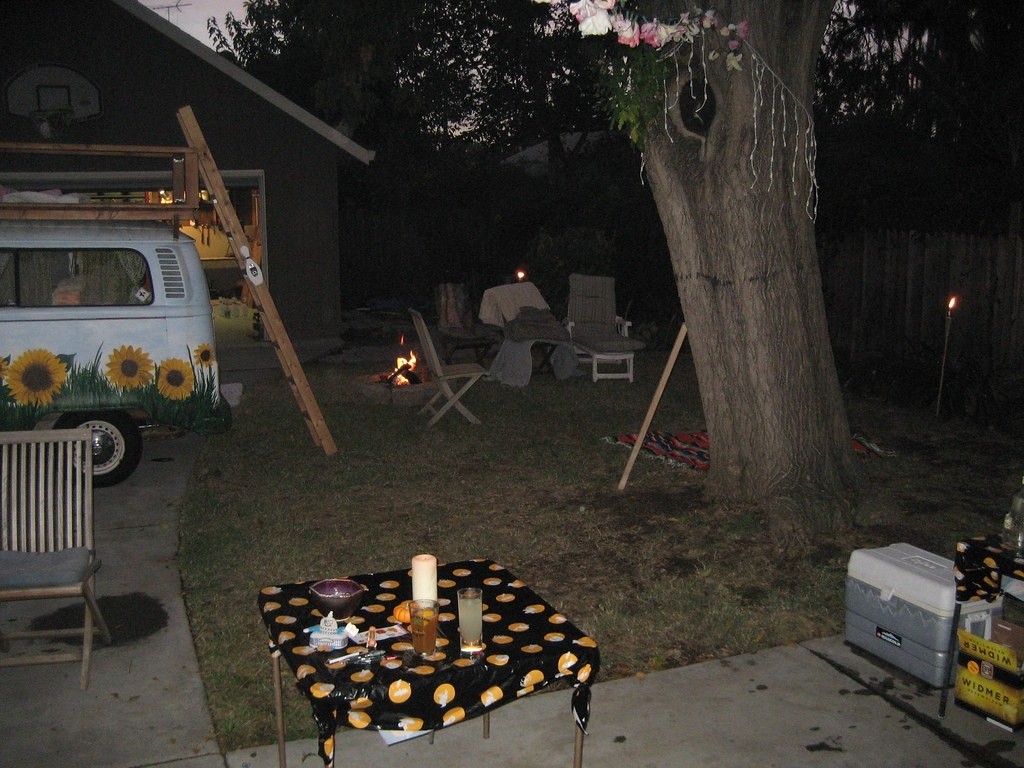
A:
0,221,230,487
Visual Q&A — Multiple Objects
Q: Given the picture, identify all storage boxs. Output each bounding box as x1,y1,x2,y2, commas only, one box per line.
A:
956,616,1024,733
844,543,1004,689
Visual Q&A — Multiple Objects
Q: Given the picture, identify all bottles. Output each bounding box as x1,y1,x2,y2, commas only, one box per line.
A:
1011,475,1024,547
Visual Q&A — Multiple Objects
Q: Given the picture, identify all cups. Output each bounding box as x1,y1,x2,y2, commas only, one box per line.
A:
409,600,440,658
1014,532,1024,558
1004,513,1012,547
457,588,484,652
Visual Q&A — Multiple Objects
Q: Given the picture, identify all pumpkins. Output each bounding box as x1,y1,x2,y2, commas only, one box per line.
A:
393,600,419,623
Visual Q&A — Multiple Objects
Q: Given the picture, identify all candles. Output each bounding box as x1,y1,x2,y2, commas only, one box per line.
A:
410,554,437,609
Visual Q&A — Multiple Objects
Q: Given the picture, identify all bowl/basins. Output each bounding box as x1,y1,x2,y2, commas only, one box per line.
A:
307,579,363,622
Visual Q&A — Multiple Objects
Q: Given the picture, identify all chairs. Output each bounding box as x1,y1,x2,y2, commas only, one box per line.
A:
562,273,646,383
490,280,570,378
433,283,499,365
0,429,112,690
408,309,487,429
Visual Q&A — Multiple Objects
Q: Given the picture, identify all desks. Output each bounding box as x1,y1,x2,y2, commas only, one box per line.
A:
937,533,1024,720
256,554,601,767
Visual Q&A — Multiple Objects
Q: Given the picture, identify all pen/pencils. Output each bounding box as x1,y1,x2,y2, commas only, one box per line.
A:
327,650,363,665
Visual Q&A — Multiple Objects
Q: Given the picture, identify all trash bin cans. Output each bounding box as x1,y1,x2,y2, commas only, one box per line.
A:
435,278,473,331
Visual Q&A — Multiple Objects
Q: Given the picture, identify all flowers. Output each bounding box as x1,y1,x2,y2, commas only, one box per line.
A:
532,0,748,74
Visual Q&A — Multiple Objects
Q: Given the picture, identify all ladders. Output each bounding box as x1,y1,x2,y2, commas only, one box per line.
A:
172,100,338,458
